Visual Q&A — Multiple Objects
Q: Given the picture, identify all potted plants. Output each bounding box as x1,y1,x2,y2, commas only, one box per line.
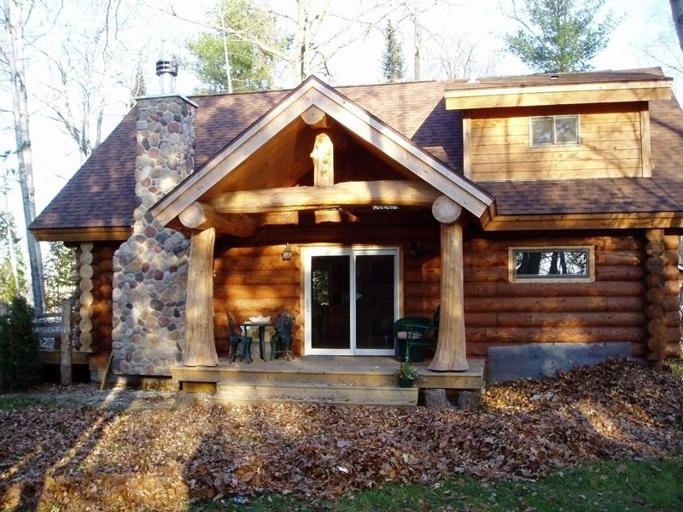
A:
395,358,425,388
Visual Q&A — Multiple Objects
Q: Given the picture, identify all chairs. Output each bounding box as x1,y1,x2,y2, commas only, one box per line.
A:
225,308,253,365
228,314,275,358
392,304,441,365
270,310,295,361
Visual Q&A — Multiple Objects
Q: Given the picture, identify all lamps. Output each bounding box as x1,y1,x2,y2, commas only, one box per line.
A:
282,242,293,260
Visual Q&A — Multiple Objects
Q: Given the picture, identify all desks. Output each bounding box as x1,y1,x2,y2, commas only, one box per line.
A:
239,323,274,362
